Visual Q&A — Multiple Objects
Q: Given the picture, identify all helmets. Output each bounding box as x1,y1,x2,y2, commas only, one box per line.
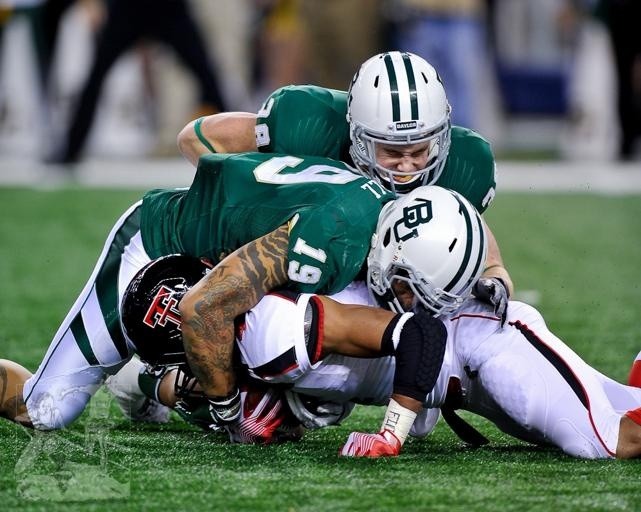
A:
119,253,225,380
344,48,454,199
364,185,490,323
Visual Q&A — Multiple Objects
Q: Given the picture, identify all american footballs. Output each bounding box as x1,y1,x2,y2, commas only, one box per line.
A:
244,383,301,429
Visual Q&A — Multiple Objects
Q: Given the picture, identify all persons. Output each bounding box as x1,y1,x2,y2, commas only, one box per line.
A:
1,0,640,174
2,48,641,464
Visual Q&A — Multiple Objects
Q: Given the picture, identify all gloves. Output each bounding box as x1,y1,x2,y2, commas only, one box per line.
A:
174,386,243,433
337,427,402,459
472,275,510,328
286,388,345,431
232,390,285,447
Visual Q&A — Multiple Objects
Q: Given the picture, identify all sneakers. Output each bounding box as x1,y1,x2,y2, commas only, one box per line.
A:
407,407,439,439
105,359,171,425
